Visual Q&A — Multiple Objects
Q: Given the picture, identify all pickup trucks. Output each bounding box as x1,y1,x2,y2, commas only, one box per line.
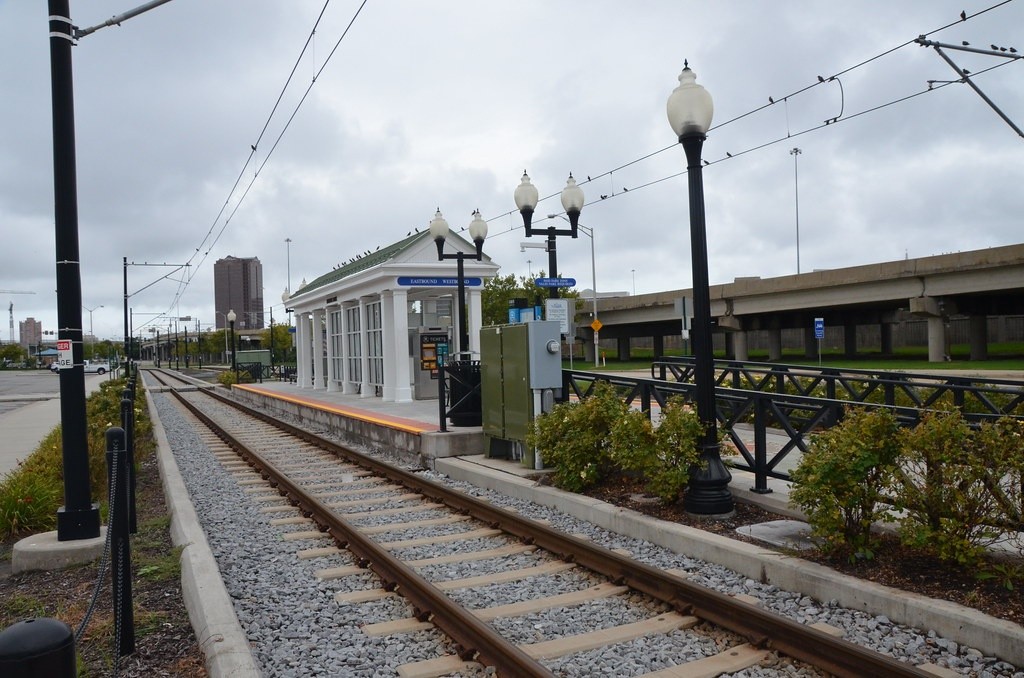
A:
84,360,110,375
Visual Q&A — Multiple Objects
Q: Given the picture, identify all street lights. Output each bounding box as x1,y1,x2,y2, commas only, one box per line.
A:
284,235,294,328
428,206,489,355
790,146,804,276
82,304,104,353
666,56,737,517
225,308,236,372
214,311,230,366
513,167,586,297
526,260,532,278
546,211,601,369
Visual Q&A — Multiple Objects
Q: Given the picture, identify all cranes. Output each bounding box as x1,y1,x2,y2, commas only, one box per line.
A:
0,290,37,346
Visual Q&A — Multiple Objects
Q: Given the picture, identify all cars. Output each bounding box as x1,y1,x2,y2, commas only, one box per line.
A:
51,361,61,374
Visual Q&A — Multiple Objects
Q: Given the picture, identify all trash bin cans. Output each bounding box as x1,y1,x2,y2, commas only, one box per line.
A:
447,360,485,428
